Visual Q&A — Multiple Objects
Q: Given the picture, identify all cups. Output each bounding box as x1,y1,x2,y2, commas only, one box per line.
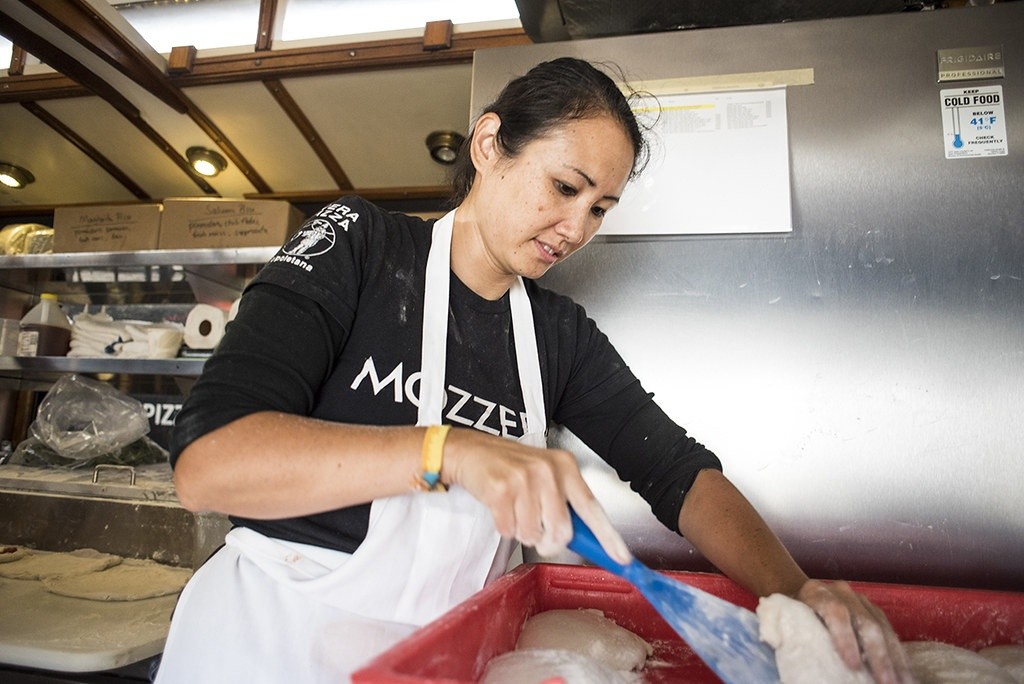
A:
147,328,182,359
0,318,21,357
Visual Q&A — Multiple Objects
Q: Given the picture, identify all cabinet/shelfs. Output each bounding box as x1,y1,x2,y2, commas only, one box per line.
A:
0,242,286,510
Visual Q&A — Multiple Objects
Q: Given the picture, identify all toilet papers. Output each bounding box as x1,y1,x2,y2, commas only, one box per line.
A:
182,302,227,348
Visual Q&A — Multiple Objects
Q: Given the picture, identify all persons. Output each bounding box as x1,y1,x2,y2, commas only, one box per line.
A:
152,56,919,684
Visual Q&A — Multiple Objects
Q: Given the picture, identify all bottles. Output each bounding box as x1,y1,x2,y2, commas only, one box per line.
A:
17,293,71,356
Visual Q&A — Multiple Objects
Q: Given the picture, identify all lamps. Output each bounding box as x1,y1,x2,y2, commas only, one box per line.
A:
425,129,467,165
185,146,228,178
0,161,36,189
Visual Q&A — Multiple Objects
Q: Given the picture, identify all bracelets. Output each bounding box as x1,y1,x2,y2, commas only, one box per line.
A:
410,426,454,494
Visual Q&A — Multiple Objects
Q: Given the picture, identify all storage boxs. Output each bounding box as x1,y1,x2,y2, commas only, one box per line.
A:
52,204,163,254
158,196,307,251
